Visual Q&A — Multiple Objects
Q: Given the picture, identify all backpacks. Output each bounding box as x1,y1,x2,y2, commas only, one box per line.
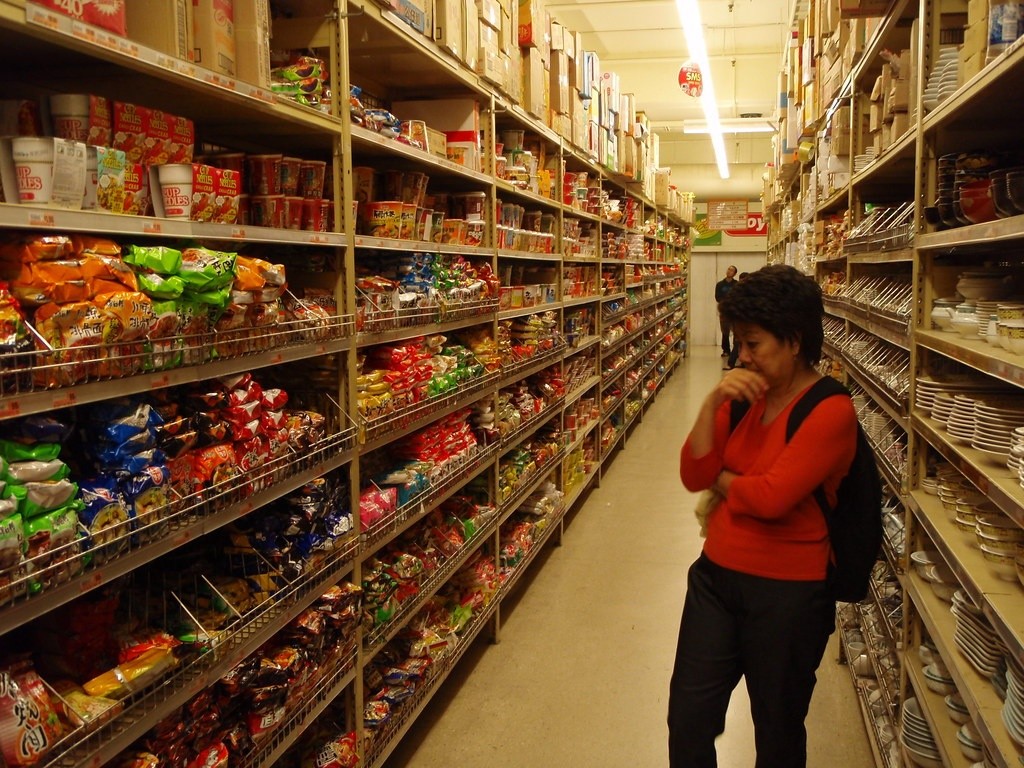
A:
728,375,882,604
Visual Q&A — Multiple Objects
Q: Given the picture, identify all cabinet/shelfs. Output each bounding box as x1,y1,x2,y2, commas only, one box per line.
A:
899,0,1024,767
835,0,918,767
765,72,850,388
348,0,691,768
0,1,366,768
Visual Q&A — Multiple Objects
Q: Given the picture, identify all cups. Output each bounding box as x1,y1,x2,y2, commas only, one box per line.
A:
889,442,909,470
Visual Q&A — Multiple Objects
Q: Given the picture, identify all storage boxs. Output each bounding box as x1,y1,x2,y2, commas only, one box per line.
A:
29,0,697,227
759,0,989,224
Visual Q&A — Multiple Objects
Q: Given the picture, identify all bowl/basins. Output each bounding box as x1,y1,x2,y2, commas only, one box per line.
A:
918,640,997,768
878,723,895,747
872,560,901,608
838,601,887,716
922,153,1024,229
897,697,943,768
910,549,955,601
987,304,1024,354
919,461,1024,591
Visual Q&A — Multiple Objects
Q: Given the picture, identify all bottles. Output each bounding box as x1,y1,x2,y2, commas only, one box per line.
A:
983,0,1024,68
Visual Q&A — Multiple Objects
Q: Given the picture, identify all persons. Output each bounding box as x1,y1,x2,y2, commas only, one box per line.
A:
666,265,859,768
715,265,752,371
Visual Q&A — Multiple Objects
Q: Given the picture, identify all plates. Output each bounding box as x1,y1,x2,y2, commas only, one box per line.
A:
997,655,1024,747
914,373,1024,488
855,395,893,445
975,301,1018,340
949,587,1002,677
922,46,961,111
